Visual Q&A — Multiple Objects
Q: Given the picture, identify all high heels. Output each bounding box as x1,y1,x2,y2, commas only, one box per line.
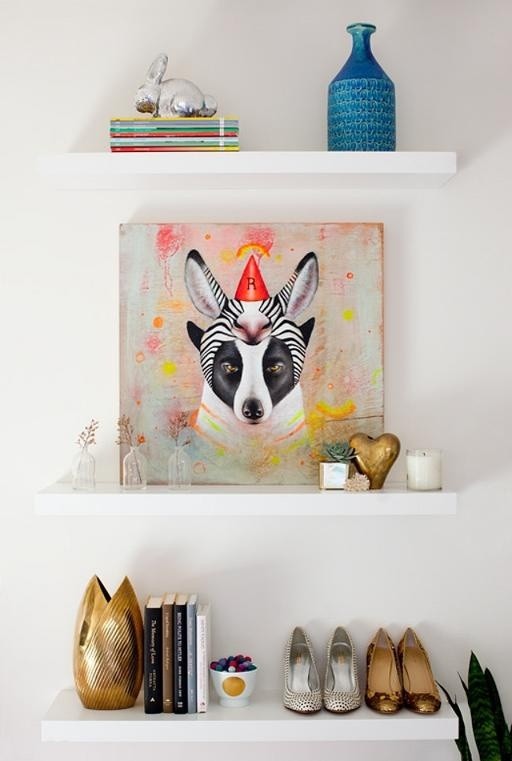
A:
282,626,322,713
322,626,362,714
365,628,404,714
397,628,441,714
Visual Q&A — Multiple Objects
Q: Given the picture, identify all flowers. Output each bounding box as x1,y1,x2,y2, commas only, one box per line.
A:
70,420,98,452
322,440,362,465
116,416,146,447
158,409,192,445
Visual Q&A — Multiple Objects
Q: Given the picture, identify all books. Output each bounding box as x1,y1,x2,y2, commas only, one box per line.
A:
142,594,162,716
170,599,187,712
162,594,174,715
104,114,241,151
183,596,195,712
195,606,214,715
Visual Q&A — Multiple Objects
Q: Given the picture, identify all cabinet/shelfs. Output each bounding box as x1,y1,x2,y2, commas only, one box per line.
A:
60,151,456,189
43,484,458,516
41,689,459,740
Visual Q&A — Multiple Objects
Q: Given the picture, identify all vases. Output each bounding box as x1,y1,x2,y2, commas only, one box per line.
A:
168,447,191,489
123,447,148,490
318,461,349,490
72,449,96,490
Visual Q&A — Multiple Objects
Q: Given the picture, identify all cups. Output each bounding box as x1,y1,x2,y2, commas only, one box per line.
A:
405,447,444,493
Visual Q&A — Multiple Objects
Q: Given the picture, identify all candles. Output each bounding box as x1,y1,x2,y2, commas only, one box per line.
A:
405,454,443,491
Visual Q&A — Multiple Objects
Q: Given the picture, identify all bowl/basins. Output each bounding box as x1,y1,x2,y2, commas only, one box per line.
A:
210,664,259,709
